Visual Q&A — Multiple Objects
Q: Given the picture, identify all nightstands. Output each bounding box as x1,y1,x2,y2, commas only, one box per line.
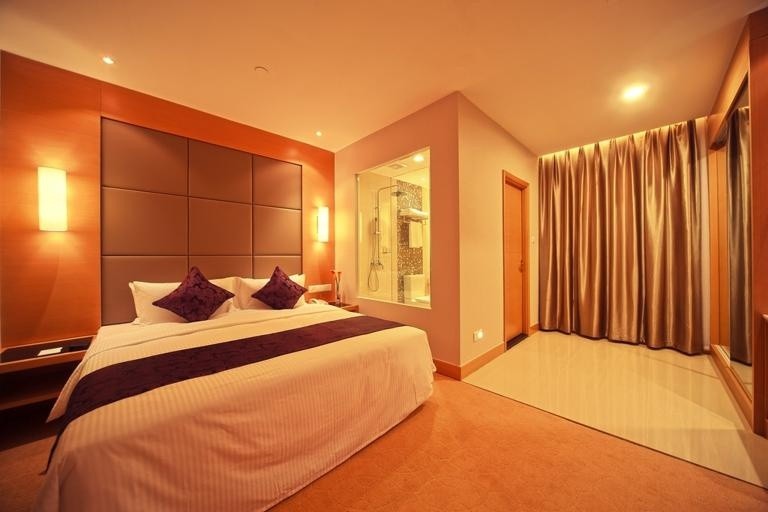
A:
0,334,93,427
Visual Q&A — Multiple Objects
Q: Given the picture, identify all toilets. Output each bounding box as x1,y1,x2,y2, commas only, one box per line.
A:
404,273,430,305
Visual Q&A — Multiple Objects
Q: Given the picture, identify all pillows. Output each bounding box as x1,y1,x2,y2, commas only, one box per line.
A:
126,265,311,326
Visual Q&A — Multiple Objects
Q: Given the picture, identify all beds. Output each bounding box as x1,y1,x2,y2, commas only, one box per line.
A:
40,318,428,512
